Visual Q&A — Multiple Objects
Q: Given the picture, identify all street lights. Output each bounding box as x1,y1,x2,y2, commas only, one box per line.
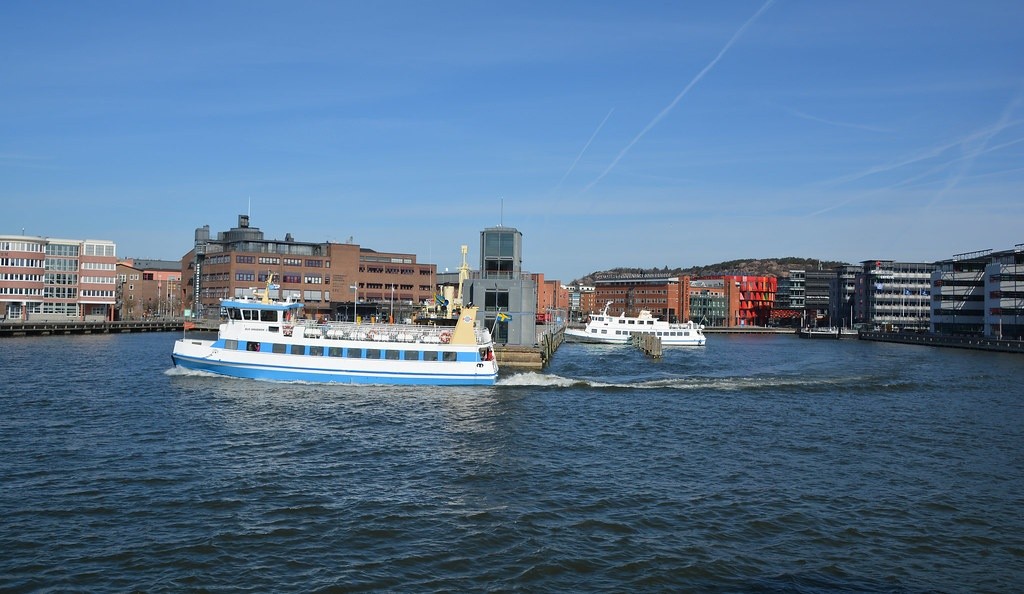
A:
391,282,394,317
353,280,357,322
841,318,844,326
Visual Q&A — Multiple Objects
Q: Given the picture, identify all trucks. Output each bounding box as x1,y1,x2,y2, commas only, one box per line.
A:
536,313,552,325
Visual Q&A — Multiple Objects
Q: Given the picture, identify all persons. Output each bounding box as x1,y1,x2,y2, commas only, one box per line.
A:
487,350,492,361
317,315,327,324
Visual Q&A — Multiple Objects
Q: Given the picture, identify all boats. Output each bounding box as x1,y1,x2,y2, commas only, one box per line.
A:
170,269,499,386
797,326,841,339
652,314,678,324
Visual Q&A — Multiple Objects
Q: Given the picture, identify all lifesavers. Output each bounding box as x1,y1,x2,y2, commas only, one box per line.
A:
440,332,451,343
367,330,377,338
284,324,293,336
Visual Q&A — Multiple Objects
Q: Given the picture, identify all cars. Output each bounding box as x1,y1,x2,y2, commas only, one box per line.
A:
562,301,707,346
840,327,847,331
577,316,582,321
892,327,900,333
873,327,881,332
569,314,574,321
153,313,160,317
579,318,587,323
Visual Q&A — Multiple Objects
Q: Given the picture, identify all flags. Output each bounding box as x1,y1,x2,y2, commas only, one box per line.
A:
255,343,260,351
249,344,253,350
185,322,194,330
921,289,928,296
497,313,512,321
905,288,912,295
434,294,449,306
704,317,708,321
